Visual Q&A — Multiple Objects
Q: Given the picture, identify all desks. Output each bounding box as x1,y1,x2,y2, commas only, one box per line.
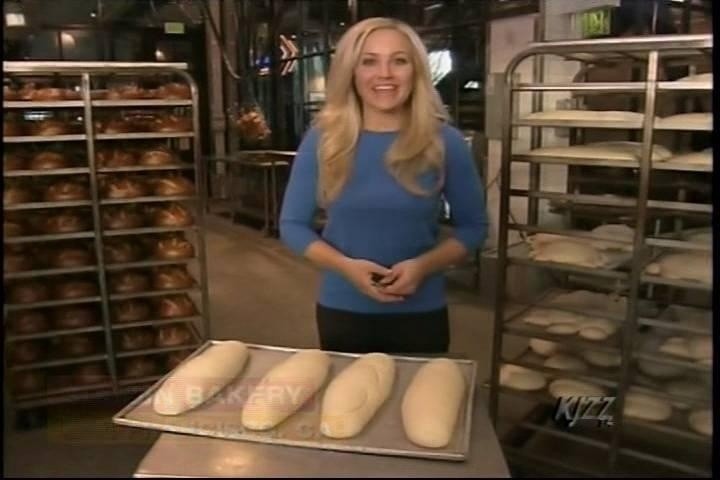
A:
132,384,511,479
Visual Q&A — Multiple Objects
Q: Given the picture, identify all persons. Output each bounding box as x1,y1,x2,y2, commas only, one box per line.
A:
279,18,488,354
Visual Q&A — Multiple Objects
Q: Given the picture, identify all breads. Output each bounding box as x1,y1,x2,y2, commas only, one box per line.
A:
401,358,465,448
319,352,395,439
0,82,193,394
499,292,713,437
154,339,249,417
234,113,272,142
241,349,332,431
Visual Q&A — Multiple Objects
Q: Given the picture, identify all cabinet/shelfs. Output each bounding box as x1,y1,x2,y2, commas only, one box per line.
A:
485,34,715,479
1,60,206,433
